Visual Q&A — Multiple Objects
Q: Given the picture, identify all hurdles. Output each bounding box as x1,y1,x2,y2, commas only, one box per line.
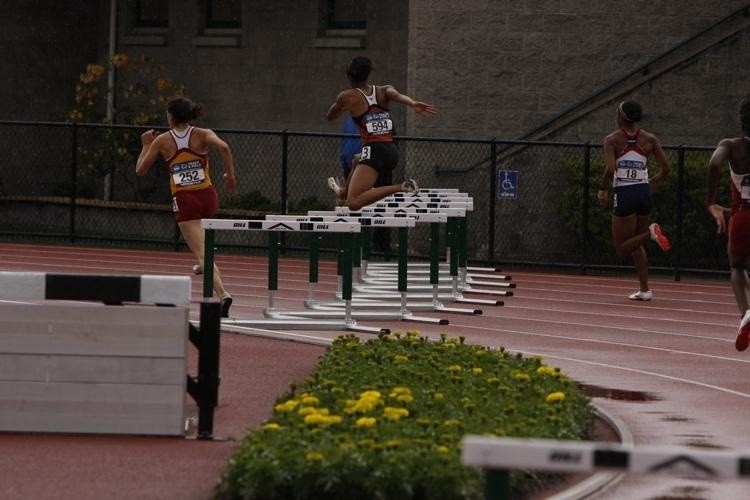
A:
459,433,749,500
190,188,516,347
0,272,222,441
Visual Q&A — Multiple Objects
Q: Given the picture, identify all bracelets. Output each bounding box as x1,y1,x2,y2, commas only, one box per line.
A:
142,140,151,146
223,173,235,179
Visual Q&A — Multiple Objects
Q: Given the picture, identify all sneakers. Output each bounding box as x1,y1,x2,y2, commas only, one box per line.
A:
629,291,652,300
328,177,345,206
401,179,418,195
650,223,671,251
193,265,202,274
221,291,232,317
736,309,750,351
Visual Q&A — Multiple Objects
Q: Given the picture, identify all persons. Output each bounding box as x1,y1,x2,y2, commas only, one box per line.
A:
325,55,437,211
135,95,239,317
340,107,399,254
705,93,749,352
596,100,673,301
